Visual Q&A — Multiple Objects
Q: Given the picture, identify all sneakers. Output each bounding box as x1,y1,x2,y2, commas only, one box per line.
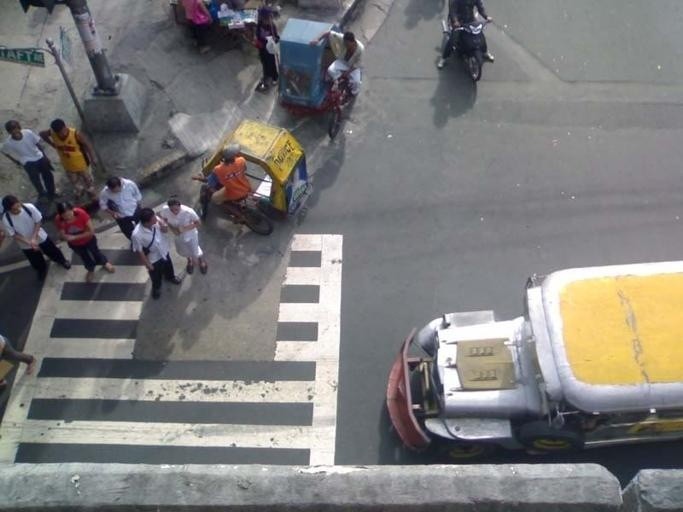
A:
37,270,48,284
34,189,100,201
186,262,195,276
102,263,117,274
151,282,162,301
23,355,39,375
437,57,447,68
198,259,208,275
60,259,72,270
165,274,182,286
484,52,494,62
85,269,97,282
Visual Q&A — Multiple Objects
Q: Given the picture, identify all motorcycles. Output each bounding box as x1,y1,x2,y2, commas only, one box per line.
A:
441,13,491,83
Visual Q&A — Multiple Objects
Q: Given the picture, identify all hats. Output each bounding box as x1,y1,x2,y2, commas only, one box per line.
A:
221,143,242,161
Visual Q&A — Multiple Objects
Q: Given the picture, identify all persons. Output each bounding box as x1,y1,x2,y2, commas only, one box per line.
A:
191,143,253,225
183,0,365,97
438,0,495,69
132,208,182,299
1,335,37,375
54,200,115,282
101,176,145,254
0,119,98,200
154,194,207,274
1,195,72,280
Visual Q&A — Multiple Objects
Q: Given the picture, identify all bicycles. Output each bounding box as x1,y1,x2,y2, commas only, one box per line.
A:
191,177,274,236
322,62,362,141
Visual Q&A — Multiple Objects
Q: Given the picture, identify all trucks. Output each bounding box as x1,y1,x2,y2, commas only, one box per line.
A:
383,259,682,462
